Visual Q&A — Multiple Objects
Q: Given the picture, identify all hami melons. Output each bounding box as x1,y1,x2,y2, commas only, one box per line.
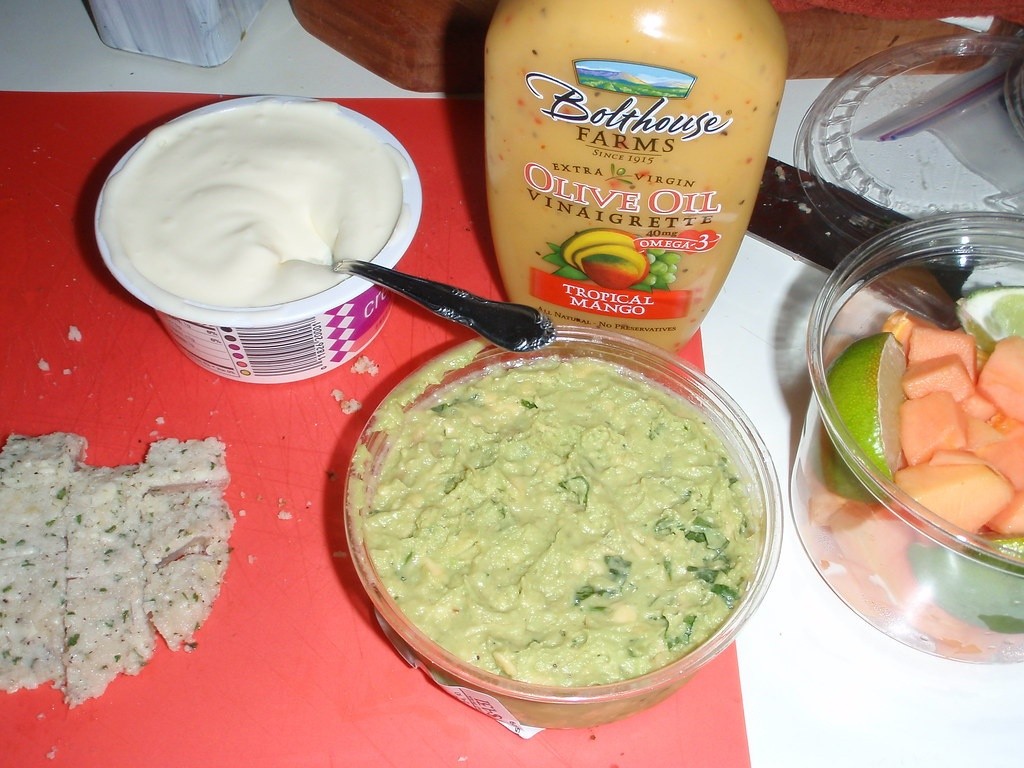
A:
811,303,1024,646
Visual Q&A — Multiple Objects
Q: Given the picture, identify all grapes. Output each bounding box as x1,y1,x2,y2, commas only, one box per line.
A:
635,245,683,292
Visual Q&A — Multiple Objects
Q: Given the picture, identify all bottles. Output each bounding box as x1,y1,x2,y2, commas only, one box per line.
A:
484,0,790,355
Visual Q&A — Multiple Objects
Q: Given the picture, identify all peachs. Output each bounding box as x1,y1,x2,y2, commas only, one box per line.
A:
559,228,649,288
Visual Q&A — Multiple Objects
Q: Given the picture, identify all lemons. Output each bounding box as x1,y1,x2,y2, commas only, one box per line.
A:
954,286,1024,352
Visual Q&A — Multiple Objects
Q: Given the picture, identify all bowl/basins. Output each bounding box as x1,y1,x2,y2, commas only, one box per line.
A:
787,210,1024,667
93,94,424,384
343,328,783,732
